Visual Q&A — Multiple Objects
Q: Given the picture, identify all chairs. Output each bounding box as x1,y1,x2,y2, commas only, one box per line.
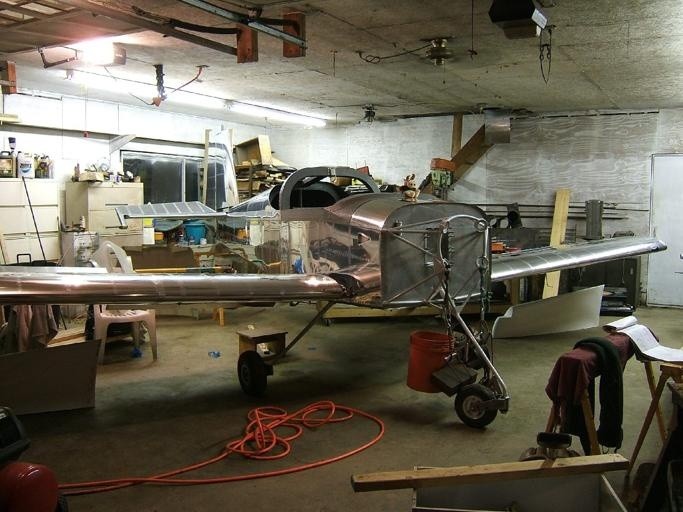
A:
88,259,158,366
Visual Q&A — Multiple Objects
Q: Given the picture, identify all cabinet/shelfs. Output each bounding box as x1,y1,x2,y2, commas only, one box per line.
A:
0,175,61,264
67,178,143,254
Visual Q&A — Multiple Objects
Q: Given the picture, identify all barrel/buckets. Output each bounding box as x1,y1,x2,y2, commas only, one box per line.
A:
184,221,207,245
17,150,35,177
407,315,455,394
0,461,57,512
1,150,17,176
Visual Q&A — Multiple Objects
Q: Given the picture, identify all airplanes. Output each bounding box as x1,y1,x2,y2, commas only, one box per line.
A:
0,166,667,427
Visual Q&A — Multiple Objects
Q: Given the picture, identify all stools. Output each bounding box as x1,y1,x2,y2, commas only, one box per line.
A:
237,326,287,362
